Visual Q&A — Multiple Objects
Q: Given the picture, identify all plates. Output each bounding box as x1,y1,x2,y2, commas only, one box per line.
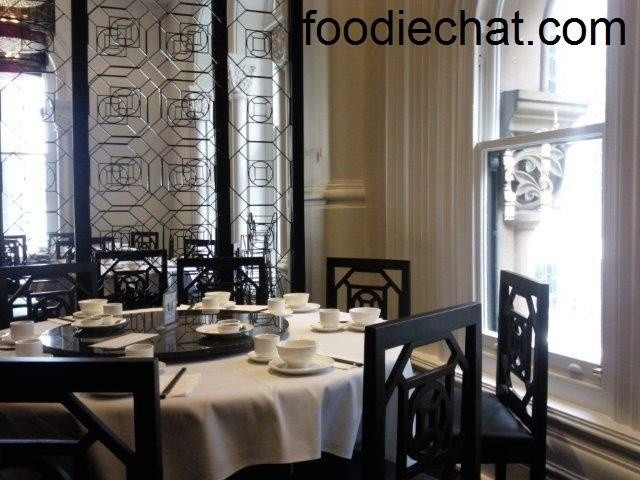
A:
70,301,367,337
247,351,336,376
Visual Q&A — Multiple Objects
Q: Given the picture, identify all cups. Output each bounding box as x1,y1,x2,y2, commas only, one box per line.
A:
102,296,341,333
125,344,156,358
255,333,279,357
10,320,45,357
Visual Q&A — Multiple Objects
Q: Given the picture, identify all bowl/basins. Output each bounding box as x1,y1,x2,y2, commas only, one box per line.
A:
77,289,384,325
127,262,141,271
276,339,319,370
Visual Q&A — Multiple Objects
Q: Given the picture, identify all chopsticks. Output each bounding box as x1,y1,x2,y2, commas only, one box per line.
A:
255,307,269,314
161,367,187,401
0,347,16,351
331,356,362,369
56,317,76,323
186,300,196,311
